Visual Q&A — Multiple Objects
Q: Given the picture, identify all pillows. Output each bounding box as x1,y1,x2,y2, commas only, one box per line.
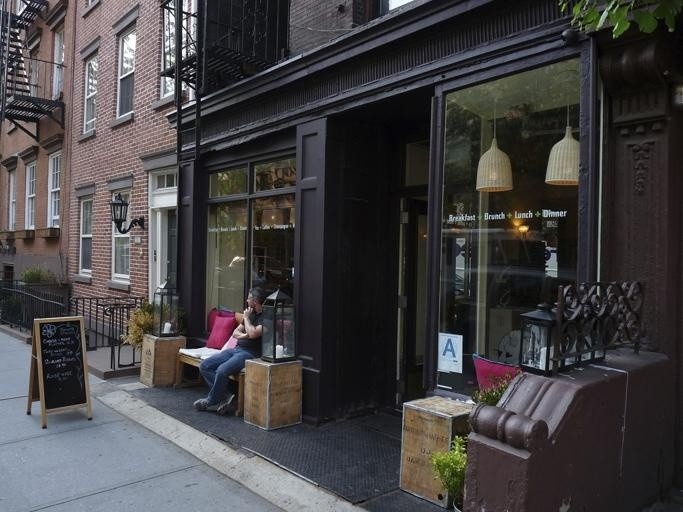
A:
205,315,235,350
216,309,234,317
205,306,219,332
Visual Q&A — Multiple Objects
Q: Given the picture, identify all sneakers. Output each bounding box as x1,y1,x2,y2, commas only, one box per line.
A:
217,392,236,414
193,398,214,411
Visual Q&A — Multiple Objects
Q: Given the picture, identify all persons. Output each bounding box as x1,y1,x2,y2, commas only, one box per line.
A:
194,286,266,416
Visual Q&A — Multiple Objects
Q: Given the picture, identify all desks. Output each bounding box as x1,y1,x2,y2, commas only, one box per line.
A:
397,395,470,509
138,333,186,389
244,356,304,432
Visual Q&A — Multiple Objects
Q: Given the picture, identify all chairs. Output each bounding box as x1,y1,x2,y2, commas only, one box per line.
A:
471,353,519,392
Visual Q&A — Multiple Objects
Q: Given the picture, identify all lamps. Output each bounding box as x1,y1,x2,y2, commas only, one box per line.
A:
152,278,181,337
259,283,298,363
473,87,512,193
516,300,606,376
541,66,579,185
106,189,144,237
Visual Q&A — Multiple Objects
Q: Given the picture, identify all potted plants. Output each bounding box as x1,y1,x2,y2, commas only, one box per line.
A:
431,434,468,512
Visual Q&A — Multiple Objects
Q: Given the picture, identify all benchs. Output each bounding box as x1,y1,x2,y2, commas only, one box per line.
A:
173,344,243,418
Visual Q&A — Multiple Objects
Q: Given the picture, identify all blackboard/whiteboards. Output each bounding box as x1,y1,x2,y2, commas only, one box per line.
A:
34,316,89,414
